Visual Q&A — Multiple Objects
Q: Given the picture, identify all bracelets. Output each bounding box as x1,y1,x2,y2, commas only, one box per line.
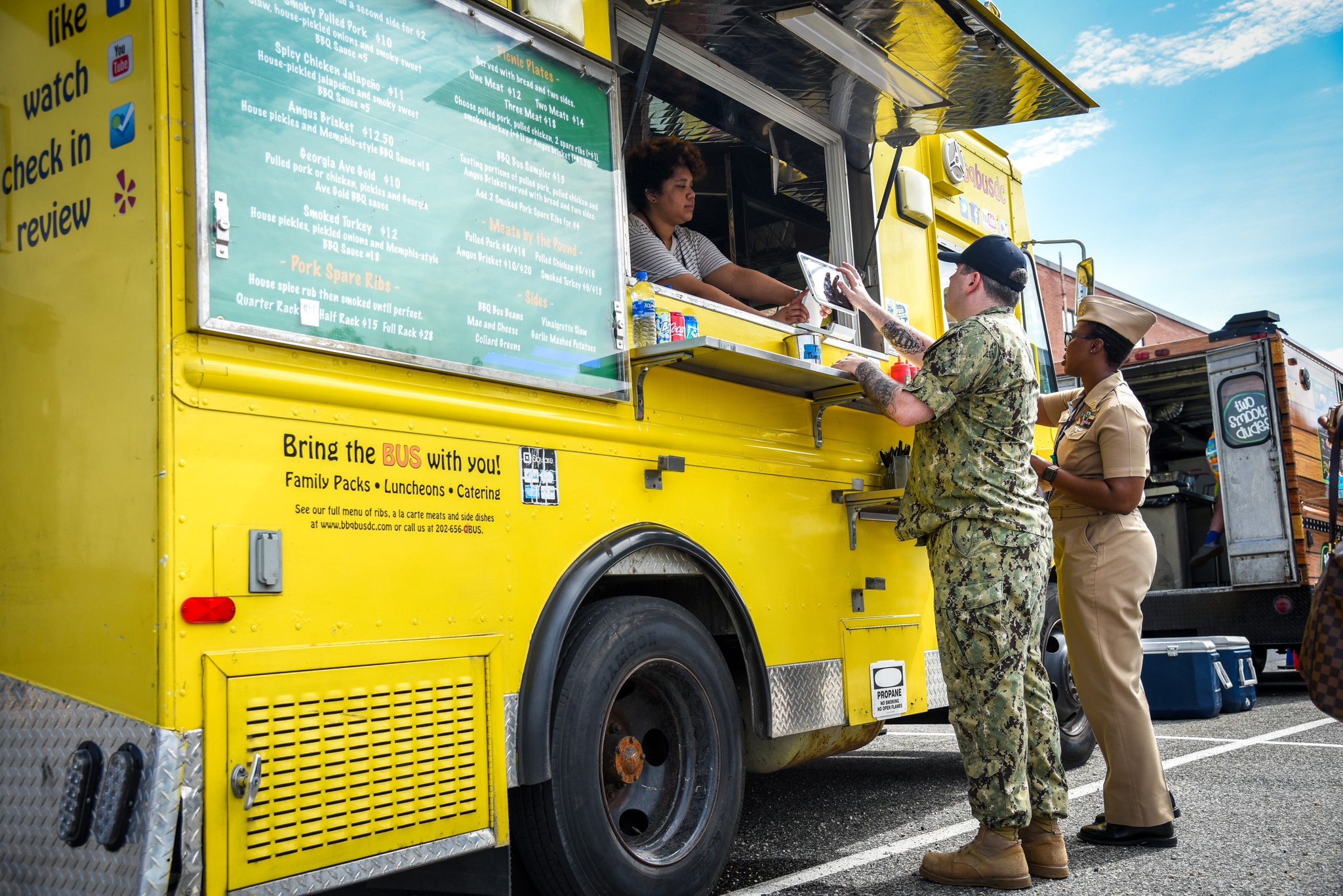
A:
792,290,803,300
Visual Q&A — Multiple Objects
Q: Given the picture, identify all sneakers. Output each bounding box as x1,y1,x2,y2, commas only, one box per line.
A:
1189,542,1222,564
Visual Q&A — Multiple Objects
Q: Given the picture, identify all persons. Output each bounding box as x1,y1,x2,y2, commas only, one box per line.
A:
1027,316,1184,848
825,234,1071,889
623,136,832,326
823,272,852,309
1189,493,1225,568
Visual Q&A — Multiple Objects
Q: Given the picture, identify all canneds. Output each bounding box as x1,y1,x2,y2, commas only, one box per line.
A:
655,307,672,344
683,315,699,339
669,311,686,341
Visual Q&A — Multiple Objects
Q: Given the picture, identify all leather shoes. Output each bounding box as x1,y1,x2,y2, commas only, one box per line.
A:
1093,791,1181,824
1076,821,1178,847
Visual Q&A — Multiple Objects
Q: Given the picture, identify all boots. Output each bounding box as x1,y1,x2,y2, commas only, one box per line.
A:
919,822,1031,889
960,818,1070,879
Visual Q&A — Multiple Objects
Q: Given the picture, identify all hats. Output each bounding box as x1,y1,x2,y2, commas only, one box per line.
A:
1077,294,1158,345
937,234,1027,292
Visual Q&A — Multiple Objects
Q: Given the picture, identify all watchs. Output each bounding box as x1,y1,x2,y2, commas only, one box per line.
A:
1041,465,1062,488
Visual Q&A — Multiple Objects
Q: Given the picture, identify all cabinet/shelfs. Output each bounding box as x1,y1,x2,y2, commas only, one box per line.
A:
629,337,906,550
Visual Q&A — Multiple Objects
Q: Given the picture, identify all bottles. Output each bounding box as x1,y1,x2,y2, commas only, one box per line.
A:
664,285,672,289
631,272,657,348
891,355,921,386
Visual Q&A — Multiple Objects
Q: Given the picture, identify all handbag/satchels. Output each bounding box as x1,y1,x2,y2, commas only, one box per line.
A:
1298,537,1343,724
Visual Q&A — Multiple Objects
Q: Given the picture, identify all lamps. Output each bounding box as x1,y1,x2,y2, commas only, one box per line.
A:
1152,402,1187,421
777,5,944,109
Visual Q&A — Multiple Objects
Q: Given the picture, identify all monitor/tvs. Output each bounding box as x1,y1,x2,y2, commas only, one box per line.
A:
796,252,857,341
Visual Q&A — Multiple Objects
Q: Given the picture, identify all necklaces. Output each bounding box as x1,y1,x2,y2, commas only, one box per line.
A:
639,207,687,270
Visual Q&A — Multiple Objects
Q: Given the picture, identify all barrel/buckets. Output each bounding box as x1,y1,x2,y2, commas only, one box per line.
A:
783,332,823,365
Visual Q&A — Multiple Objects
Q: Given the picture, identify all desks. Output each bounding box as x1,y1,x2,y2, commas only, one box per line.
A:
1144,484,1221,585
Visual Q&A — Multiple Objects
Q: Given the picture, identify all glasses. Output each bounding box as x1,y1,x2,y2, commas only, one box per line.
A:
1065,332,1095,348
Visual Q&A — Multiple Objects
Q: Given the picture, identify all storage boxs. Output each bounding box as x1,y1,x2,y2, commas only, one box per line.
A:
1141,635,1259,712
1143,641,1233,719
